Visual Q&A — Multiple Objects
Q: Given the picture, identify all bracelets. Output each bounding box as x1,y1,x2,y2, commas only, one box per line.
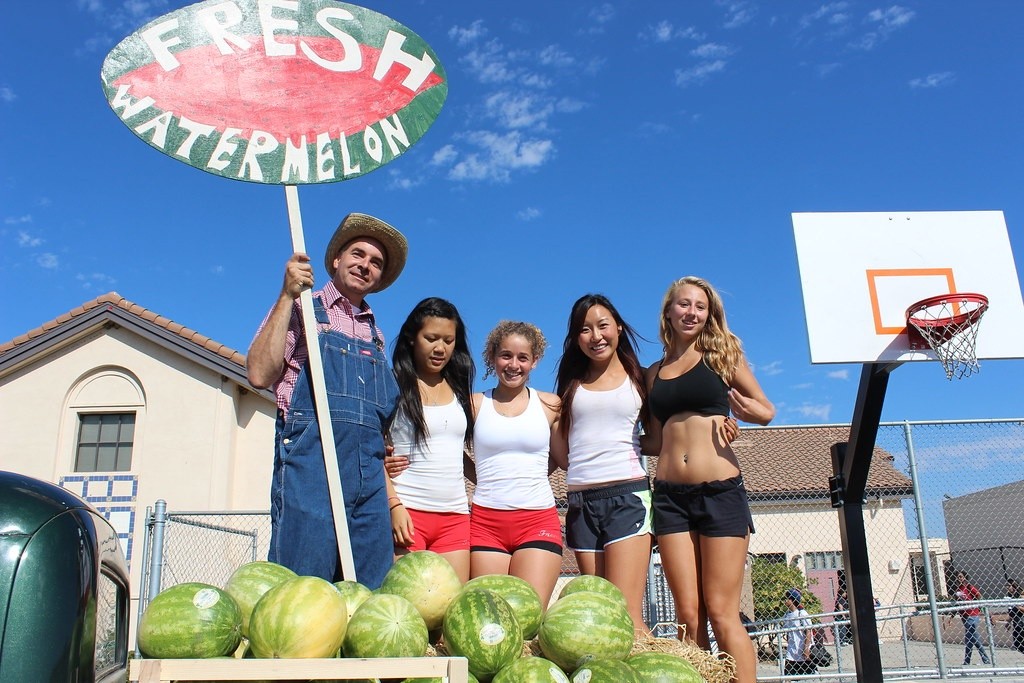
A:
387,495,401,502
389,503,403,511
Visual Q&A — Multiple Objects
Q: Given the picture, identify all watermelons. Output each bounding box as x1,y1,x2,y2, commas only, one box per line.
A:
137,551,706,682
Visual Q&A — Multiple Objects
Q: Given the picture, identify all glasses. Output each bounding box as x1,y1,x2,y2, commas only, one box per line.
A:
957,576,964,578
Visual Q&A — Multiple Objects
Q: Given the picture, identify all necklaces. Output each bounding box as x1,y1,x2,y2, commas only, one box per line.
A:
495,381,527,417
412,365,442,406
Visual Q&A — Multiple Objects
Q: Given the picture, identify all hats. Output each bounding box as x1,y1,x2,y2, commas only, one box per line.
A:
786,589,805,609
325,212,409,293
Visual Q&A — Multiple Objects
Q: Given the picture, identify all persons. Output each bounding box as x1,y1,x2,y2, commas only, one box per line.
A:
1004,577,1024,656
377,296,477,592
247,210,409,590
948,570,991,665
639,275,775,683
546,292,742,637
381,317,570,612
832,587,885,646
782,590,821,681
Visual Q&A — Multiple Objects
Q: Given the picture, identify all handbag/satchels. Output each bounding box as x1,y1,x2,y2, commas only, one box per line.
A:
809,641,834,667
